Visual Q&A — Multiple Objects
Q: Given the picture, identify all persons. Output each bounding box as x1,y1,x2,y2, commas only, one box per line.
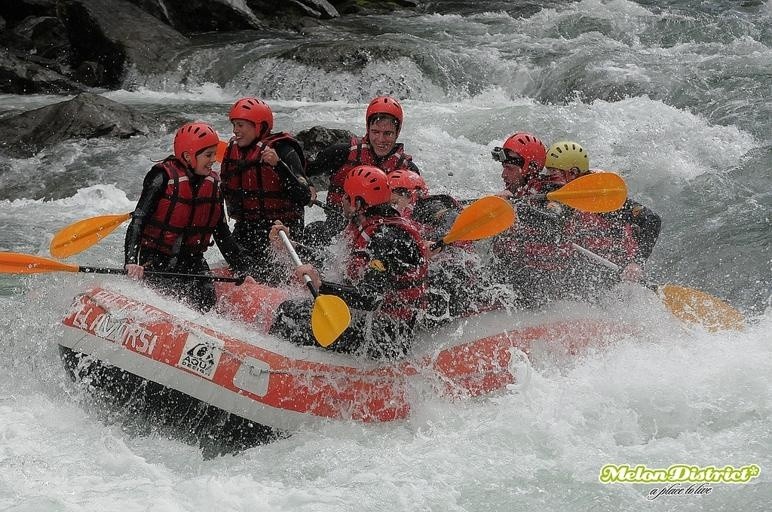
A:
122,121,263,317
219,97,663,363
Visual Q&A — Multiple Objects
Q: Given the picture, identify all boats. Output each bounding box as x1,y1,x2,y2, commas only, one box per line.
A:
57,195,666,438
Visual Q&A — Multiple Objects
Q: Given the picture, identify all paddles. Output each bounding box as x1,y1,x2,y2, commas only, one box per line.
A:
0,252,253,283
50,212,134,259
276,229,350,349
570,243,745,336
456,172,629,214
427,196,517,248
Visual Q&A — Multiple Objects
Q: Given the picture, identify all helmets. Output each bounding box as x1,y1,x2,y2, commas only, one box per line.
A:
387,170,428,205
174,123,218,169
504,132,546,176
366,96,403,125
545,142,589,175
344,165,391,207
229,98,273,138
